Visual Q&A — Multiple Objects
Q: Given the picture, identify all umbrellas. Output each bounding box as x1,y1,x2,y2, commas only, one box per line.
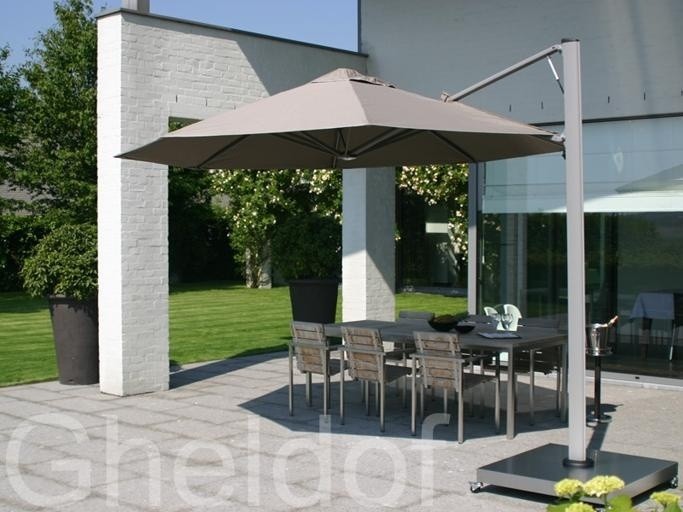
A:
114,68,567,169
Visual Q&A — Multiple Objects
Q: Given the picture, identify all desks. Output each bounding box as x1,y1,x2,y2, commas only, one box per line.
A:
629,290,683,362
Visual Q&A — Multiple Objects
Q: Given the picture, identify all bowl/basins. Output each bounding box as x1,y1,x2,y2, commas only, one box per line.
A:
428,320,457,332
454,325,475,334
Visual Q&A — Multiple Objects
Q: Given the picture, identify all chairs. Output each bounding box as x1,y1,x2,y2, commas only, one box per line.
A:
285,304,562,442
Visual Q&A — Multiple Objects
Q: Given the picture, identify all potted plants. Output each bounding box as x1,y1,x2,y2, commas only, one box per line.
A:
17,222,98,385
269,213,342,323
